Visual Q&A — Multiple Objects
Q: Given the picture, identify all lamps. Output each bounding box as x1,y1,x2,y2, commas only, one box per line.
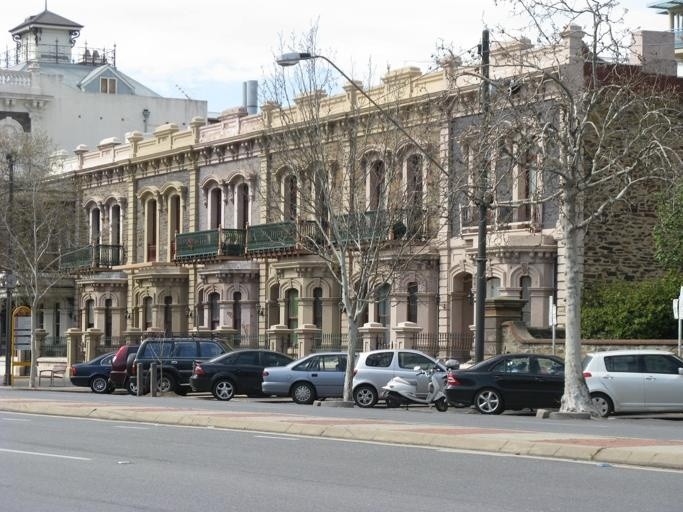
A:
467,291,474,305
124,310,130,319
67,311,79,322
256,302,264,316
338,299,346,313
184,305,192,318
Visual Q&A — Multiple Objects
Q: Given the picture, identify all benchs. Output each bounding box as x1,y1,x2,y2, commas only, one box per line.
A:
39,369,65,387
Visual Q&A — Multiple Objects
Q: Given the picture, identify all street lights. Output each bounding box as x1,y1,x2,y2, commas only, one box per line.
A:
275,28,490,364
